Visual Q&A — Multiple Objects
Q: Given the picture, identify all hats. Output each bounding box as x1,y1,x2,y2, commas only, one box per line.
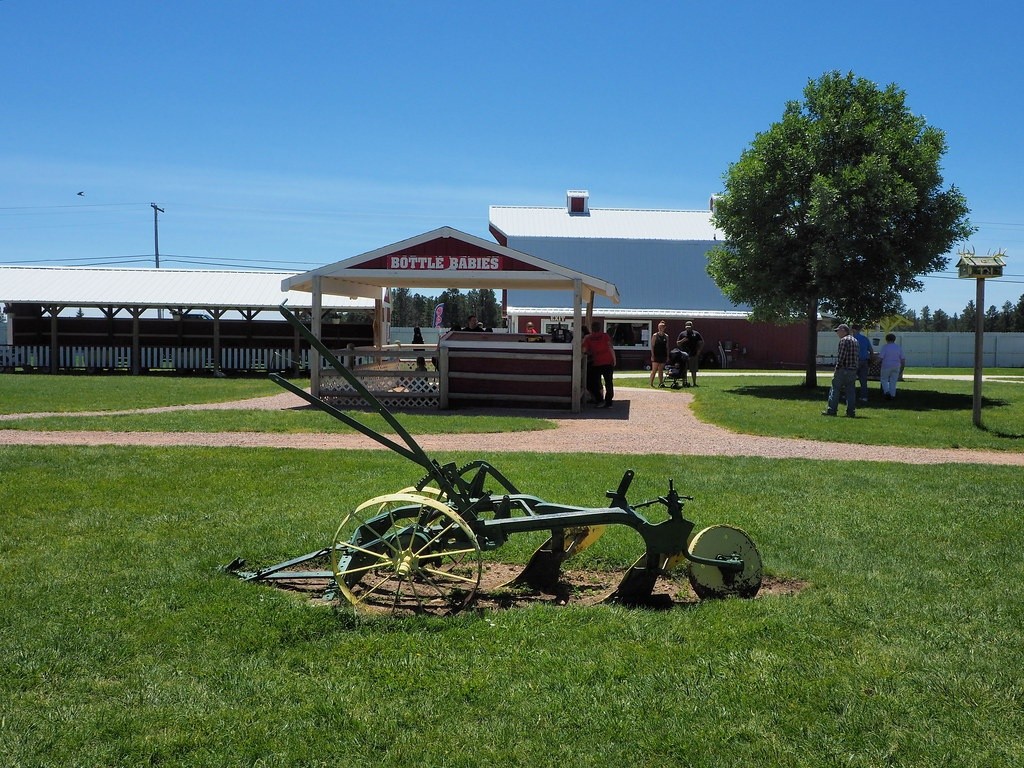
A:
685,321,693,329
833,324,849,331
852,324,861,330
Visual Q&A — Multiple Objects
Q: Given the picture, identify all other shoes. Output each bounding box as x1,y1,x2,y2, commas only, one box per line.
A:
882,394,888,398
659,383,699,387
846,414,855,418
859,397,868,401
822,411,837,416
890,397,895,401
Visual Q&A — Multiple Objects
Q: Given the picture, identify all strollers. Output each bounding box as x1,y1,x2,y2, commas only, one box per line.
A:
658,348,692,389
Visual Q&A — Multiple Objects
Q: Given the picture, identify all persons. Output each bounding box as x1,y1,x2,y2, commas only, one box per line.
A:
580,325,603,404
820,324,860,418
411,327,426,352
461,315,485,332
665,363,682,376
872,333,906,402
581,322,617,409
649,321,669,388
849,324,875,404
415,357,429,392
677,321,705,387
525,322,538,334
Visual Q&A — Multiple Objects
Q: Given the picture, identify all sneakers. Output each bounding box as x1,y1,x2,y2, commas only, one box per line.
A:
596,400,605,408
604,404,613,408
